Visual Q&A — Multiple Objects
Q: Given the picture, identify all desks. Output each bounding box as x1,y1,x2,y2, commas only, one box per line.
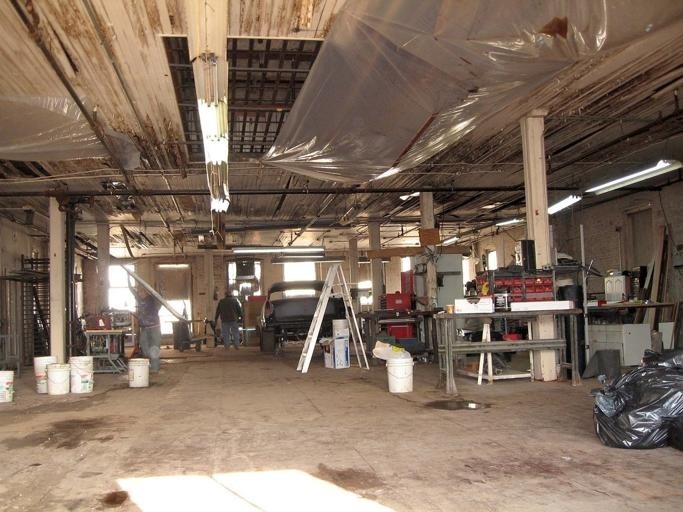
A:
431,300,675,395
82,330,128,376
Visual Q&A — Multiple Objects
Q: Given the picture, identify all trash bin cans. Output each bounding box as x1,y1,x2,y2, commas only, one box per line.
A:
173,322,190,349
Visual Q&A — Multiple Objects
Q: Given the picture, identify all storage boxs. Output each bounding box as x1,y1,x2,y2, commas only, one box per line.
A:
324,337,350,370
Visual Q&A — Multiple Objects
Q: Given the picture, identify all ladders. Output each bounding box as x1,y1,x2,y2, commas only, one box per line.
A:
296,262,370,373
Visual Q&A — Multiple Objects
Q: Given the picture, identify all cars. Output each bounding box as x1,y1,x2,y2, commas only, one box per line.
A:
256,281,345,352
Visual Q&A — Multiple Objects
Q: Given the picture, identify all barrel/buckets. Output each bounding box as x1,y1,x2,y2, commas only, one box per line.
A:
70,356,93,394
333,319,348,335
33,356,58,394
0,370,14,403
386,358,414,394
127,358,150,388
45,363,70,395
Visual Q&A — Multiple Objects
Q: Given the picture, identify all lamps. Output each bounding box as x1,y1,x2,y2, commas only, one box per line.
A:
545,157,683,215
231,246,326,257
196,53,240,215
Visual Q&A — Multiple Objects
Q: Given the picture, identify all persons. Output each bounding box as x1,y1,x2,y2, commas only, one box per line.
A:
214,287,244,350
123,271,163,375
